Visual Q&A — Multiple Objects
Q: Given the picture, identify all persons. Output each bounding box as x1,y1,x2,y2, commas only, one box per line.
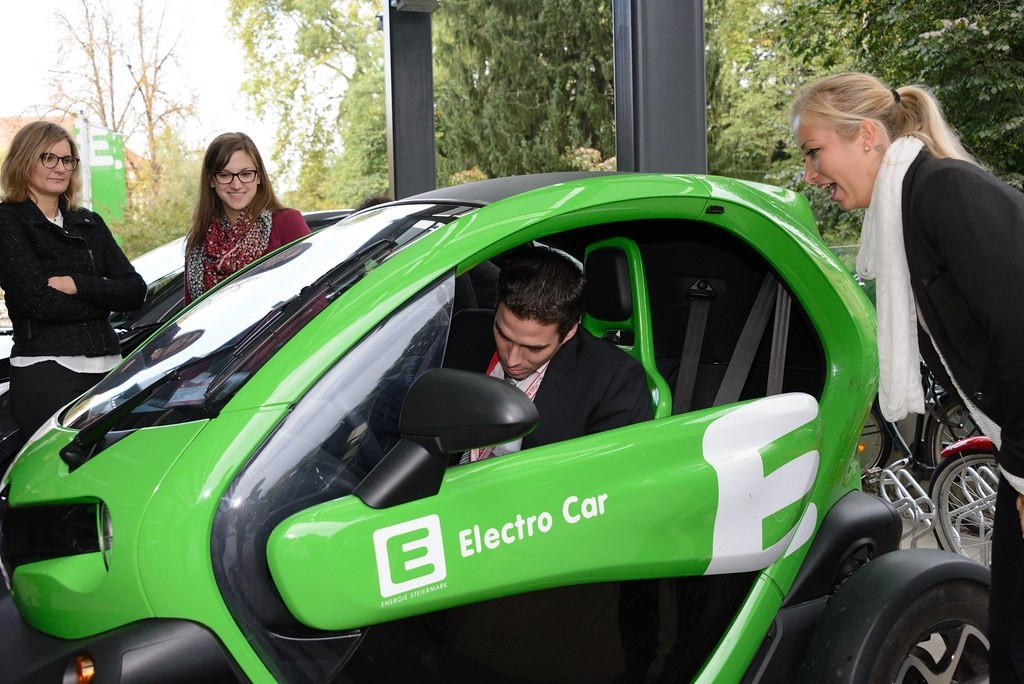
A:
352,247,653,684
788,73,1022,684
355,196,394,213
185,133,311,311
0,121,147,441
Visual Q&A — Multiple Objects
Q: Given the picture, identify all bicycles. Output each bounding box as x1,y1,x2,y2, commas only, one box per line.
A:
860,360,986,488
864,437,998,569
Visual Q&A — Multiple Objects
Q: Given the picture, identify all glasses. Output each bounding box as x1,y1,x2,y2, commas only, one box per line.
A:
212,170,258,184
39,152,80,171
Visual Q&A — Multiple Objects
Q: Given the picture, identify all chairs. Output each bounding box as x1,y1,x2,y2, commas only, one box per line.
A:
578,234,674,418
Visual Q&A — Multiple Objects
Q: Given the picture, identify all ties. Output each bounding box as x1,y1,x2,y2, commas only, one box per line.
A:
458,375,521,466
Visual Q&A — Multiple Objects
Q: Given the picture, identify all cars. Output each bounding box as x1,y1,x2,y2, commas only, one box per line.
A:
0,172,993,684
0,209,359,478
